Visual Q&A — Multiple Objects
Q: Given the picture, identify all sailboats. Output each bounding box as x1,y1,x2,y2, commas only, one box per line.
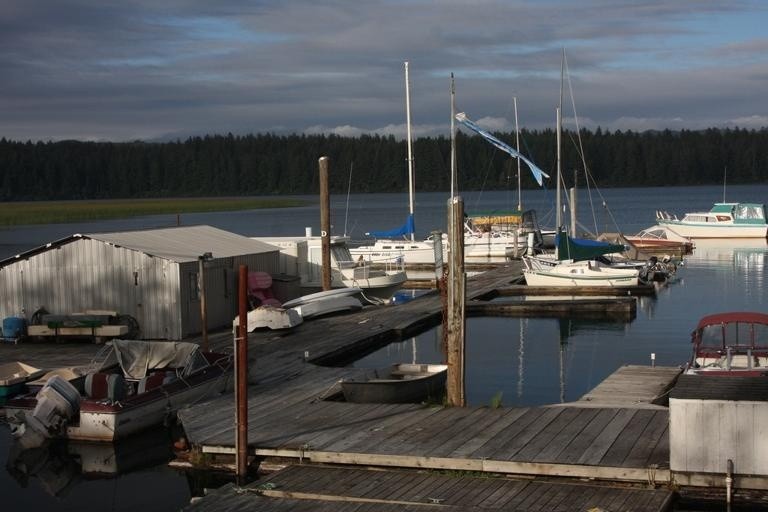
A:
463,47,694,287
347,60,449,264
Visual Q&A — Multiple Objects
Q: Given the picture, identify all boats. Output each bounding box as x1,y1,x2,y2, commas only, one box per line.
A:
247,231,408,305
5,338,256,450
341,363,448,404
231,306,303,332
288,296,365,320
281,288,364,303
0,361,44,405
9,424,185,503
684,312,768,377
655,167,768,238
